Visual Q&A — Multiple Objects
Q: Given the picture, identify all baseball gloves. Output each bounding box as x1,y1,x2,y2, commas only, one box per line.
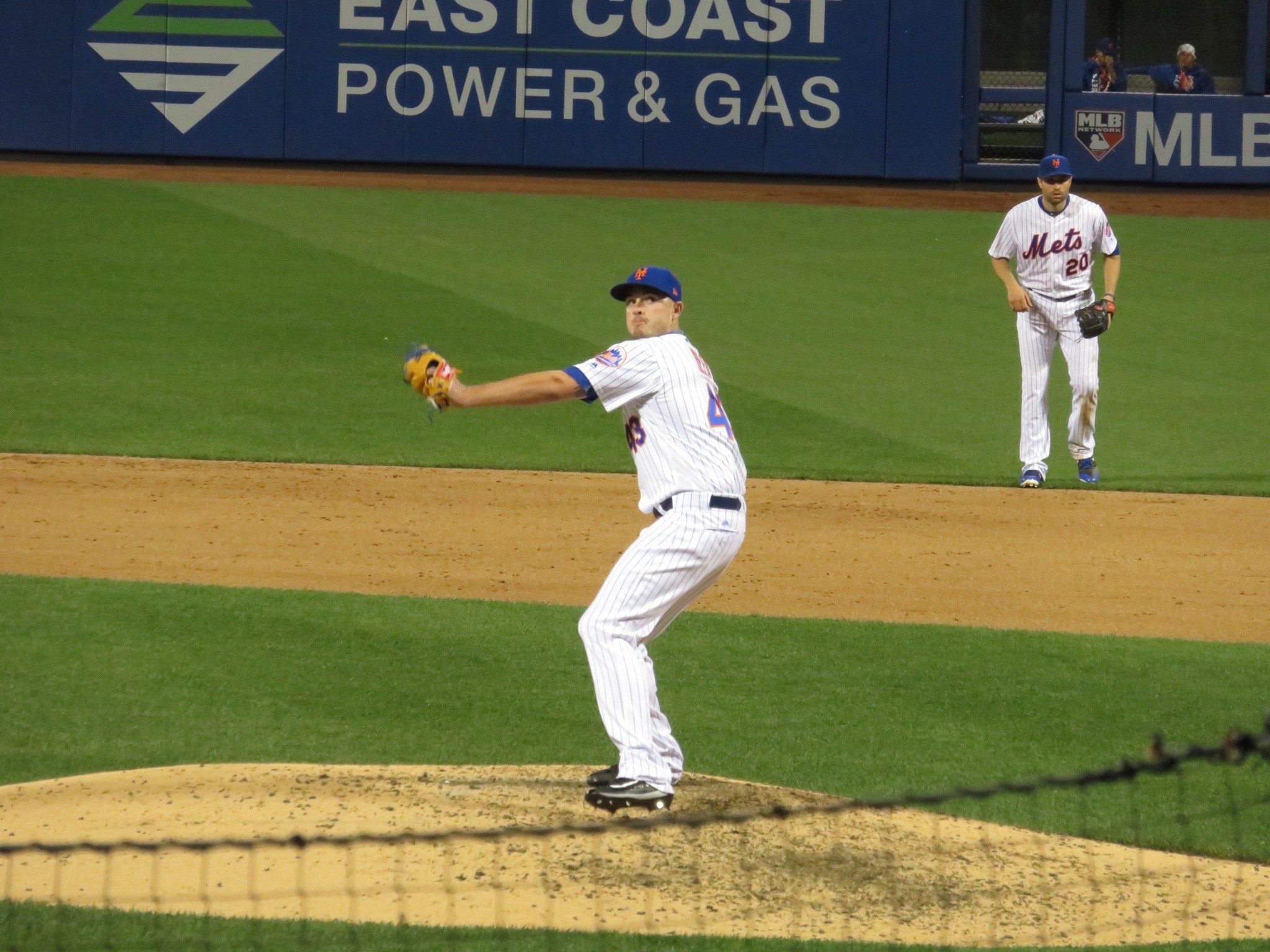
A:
1074,299,1115,338
403,346,455,410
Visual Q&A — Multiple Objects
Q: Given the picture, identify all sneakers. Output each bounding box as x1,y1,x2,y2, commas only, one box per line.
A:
583,776,673,816
586,763,627,787
1077,457,1101,484
1020,470,1043,490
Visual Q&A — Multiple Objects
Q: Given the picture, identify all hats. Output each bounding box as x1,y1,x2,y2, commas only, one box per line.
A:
609,265,683,302
1096,37,1119,56
1037,152,1075,180
1175,44,1197,59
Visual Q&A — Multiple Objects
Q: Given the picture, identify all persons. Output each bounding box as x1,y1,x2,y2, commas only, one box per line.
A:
990,155,1120,488
1080,31,1128,92
404,268,747,812
1147,44,1212,93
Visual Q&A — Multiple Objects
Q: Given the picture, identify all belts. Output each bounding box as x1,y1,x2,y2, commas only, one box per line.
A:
650,495,743,520
1024,286,1076,302
